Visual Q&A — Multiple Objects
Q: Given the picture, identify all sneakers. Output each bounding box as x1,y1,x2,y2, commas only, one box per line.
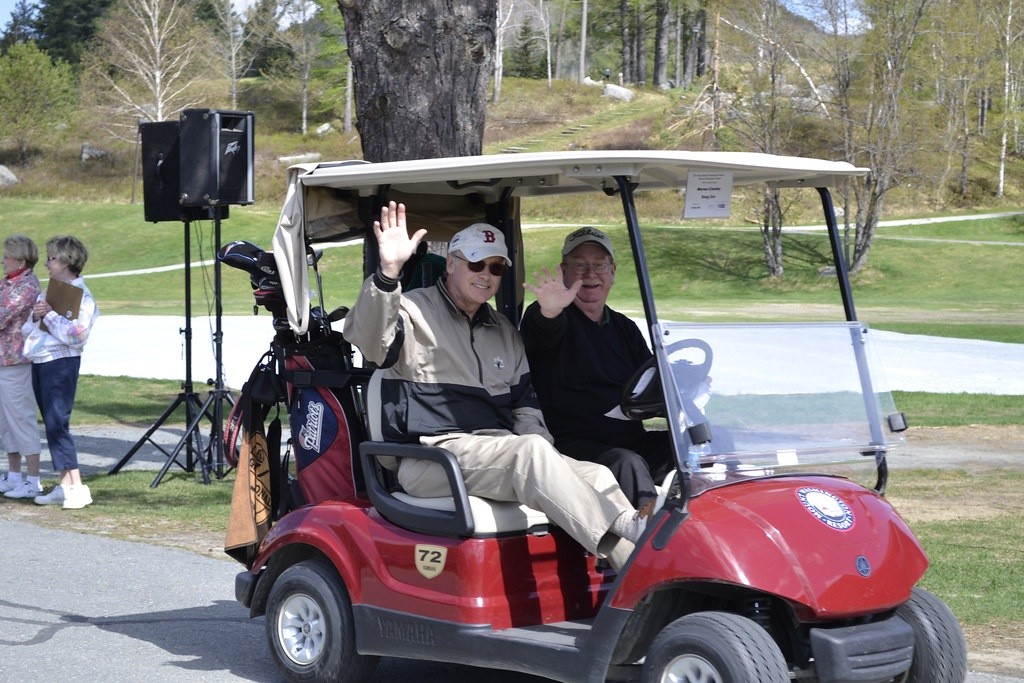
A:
34,485,64,505
0,476,23,492
632,468,681,547
4,481,43,498
62,485,93,509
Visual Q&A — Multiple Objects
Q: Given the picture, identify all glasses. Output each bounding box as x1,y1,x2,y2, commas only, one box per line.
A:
561,262,612,275
452,255,507,276
46,255,56,262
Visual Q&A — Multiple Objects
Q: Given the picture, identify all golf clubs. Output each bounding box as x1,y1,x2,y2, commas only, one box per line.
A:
311,305,350,336
306,250,323,265
303,242,325,321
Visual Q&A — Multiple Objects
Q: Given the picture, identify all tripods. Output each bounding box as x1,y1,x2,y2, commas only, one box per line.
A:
108,221,238,489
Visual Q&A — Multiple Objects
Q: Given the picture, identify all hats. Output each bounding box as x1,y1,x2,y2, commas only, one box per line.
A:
448,223,512,267
562,227,614,261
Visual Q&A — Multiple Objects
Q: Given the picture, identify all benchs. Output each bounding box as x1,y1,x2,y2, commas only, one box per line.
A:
358,369,558,539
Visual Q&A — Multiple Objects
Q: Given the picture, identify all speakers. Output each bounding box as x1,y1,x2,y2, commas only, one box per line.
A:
140,120,229,224
178,108,254,205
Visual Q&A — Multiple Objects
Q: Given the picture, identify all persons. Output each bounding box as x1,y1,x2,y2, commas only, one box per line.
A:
522,225,736,511
343,200,679,576
0,236,44,498
20,235,98,509
